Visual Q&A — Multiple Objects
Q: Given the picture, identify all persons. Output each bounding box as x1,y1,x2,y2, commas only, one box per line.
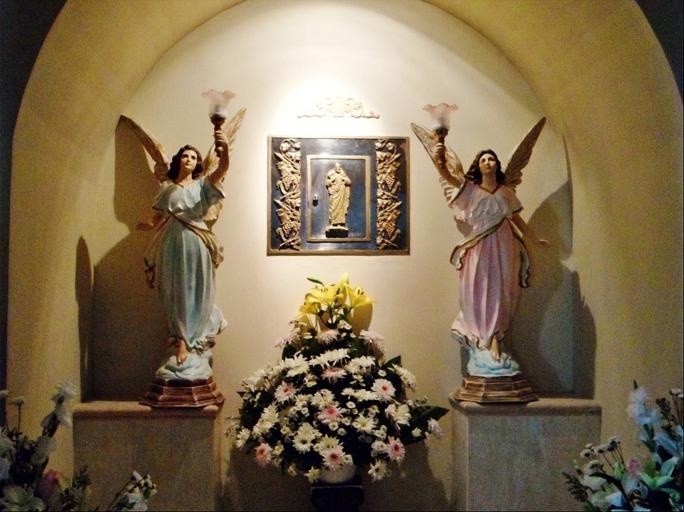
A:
435,141,551,366
134,130,230,366
327,161,352,226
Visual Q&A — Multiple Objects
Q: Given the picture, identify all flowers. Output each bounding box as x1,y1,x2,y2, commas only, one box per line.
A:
0,377,158,512
222,272,450,482
559,378,684,511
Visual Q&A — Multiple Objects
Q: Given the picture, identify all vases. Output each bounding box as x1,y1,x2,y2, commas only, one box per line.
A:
309,483,364,512
